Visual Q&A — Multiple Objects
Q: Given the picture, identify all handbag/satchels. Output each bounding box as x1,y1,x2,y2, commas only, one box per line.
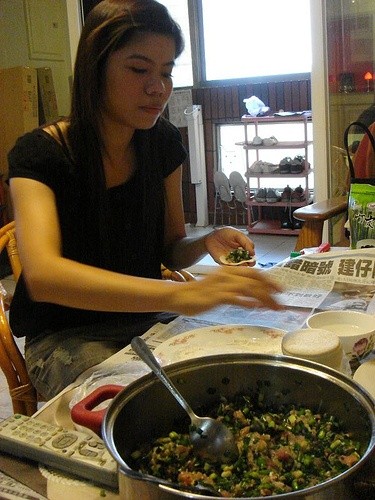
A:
347,177,375,250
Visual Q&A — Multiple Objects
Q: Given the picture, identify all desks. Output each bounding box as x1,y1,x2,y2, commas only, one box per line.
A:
0,248,375,500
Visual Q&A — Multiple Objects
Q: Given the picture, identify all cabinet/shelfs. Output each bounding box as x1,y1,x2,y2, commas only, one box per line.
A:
241,110,314,235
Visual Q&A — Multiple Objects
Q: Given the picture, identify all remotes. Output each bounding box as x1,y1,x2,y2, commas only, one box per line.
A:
0,413,122,489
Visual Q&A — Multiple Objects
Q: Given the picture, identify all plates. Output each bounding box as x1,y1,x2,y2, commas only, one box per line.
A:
152,324,289,368
353,358,375,399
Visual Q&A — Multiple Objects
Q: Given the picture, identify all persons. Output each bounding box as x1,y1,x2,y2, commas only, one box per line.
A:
8,0,285,402
344,121,375,191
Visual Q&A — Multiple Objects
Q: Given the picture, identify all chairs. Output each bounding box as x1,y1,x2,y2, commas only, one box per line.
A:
212,171,254,227
0,220,196,416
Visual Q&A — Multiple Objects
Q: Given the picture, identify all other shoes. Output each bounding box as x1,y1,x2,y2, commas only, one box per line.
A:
289,156,310,174
253,135,262,145
279,158,291,173
264,136,278,146
264,162,278,173
281,217,288,228
213,170,232,201
293,218,300,229
254,164,262,174
229,171,247,202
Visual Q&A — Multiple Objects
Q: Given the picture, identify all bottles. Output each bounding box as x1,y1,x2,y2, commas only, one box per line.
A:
281,328,343,370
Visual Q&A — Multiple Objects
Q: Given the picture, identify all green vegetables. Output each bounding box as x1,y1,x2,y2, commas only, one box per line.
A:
127,377,364,497
230,249,252,263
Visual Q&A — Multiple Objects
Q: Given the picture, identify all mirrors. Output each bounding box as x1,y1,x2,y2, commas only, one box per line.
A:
0,0,84,126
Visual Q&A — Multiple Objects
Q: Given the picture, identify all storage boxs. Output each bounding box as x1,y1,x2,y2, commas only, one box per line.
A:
39,67,59,122
0,66,40,220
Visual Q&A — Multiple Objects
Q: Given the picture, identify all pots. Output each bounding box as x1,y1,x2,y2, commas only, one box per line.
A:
70,353,375,500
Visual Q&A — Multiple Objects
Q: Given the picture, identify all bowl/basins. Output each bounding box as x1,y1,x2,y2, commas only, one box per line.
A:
306,310,375,366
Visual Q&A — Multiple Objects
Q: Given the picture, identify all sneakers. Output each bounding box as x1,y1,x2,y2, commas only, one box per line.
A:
291,186,311,202
256,188,266,202
282,186,293,202
266,189,282,202
